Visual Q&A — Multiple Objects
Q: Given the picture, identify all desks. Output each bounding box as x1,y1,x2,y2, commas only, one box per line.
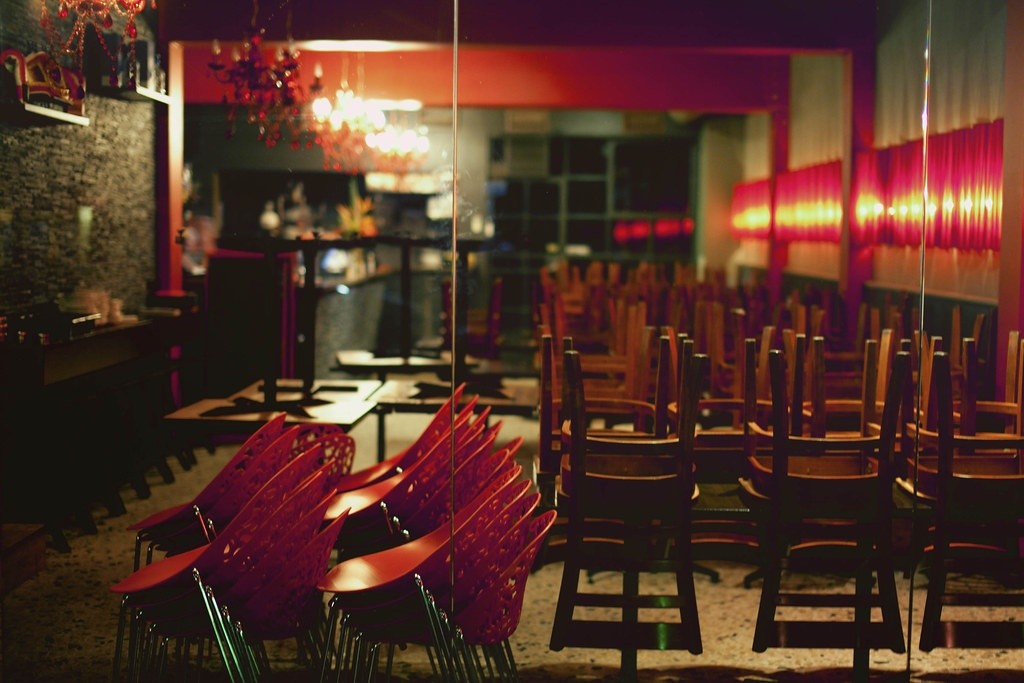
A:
227,378,382,402
367,379,541,461
159,398,378,465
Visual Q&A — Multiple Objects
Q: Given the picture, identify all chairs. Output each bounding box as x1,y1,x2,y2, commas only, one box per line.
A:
108,258,1024,682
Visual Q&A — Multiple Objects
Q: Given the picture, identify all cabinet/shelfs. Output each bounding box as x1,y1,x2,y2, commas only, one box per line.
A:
20,83,171,126
489,130,697,350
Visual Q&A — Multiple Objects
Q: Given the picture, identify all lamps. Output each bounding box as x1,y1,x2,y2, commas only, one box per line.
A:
198,0,438,192
39,0,159,89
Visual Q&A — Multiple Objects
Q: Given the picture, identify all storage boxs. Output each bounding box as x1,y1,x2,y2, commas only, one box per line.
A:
503,107,555,132
